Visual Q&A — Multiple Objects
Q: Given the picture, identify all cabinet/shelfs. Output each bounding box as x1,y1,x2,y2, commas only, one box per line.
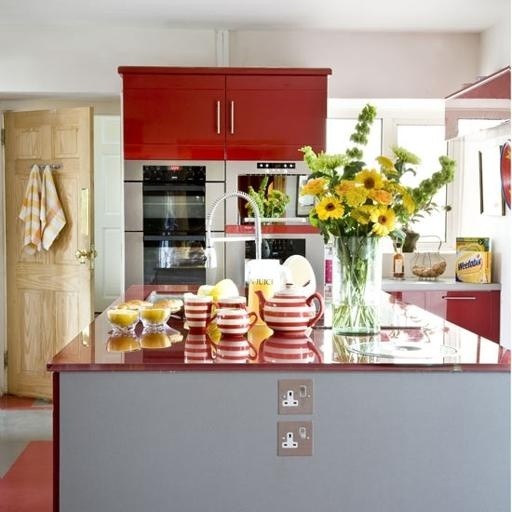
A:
382,281,499,347
117,65,333,161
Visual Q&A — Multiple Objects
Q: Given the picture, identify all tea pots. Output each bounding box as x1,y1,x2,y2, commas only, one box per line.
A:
262,336,323,363
253,290,323,333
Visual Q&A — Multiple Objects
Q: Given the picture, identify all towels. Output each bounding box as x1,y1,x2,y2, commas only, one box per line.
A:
17,164,67,255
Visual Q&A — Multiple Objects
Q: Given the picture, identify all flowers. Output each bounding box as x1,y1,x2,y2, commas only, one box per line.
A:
299,105,456,332
244,177,290,226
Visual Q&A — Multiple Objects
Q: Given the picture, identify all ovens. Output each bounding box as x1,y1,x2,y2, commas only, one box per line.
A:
125,159,224,291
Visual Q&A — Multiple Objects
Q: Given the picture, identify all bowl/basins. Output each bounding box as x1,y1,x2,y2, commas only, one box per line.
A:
103,336,172,354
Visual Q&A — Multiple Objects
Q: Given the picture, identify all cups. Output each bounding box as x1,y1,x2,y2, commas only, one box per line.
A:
105,307,167,335
183,334,258,362
184,297,257,338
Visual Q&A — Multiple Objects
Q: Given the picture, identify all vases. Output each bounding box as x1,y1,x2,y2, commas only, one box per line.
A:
330,236,379,335
262,239,271,259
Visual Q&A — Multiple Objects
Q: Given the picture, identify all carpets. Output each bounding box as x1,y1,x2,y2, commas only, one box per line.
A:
0,392,53,511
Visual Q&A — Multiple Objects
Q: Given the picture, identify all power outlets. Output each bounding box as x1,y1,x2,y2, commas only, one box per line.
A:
278,379,314,457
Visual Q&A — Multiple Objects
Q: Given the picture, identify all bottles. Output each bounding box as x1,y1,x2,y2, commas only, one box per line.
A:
393,241,404,278
324,245,338,286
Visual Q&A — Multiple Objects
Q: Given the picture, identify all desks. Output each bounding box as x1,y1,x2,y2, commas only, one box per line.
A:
47,283,511,512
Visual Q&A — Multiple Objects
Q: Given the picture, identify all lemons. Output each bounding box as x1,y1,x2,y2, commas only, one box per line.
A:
141,310,166,324
105,336,140,353
139,333,170,348
106,309,136,328
